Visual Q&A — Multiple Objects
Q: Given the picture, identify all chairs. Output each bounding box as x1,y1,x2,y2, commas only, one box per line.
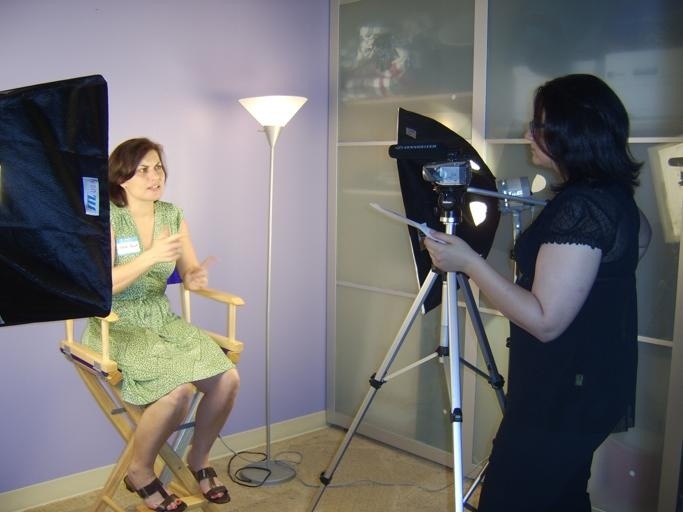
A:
58,282,244,511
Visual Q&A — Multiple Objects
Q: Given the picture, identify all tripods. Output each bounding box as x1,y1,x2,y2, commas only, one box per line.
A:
306,194,511,512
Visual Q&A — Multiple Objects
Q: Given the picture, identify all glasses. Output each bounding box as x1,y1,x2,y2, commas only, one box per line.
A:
528,120,546,134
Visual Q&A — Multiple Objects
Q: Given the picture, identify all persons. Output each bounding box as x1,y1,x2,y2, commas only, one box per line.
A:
423,73,642,510
80,136,241,511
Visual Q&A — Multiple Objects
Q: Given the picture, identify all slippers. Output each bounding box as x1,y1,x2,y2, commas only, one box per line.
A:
123,473,187,512
188,466,230,504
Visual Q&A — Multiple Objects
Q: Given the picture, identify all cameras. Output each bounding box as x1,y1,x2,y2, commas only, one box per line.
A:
388,141,473,193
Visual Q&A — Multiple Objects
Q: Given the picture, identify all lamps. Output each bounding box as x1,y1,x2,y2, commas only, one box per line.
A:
237,94,308,485
